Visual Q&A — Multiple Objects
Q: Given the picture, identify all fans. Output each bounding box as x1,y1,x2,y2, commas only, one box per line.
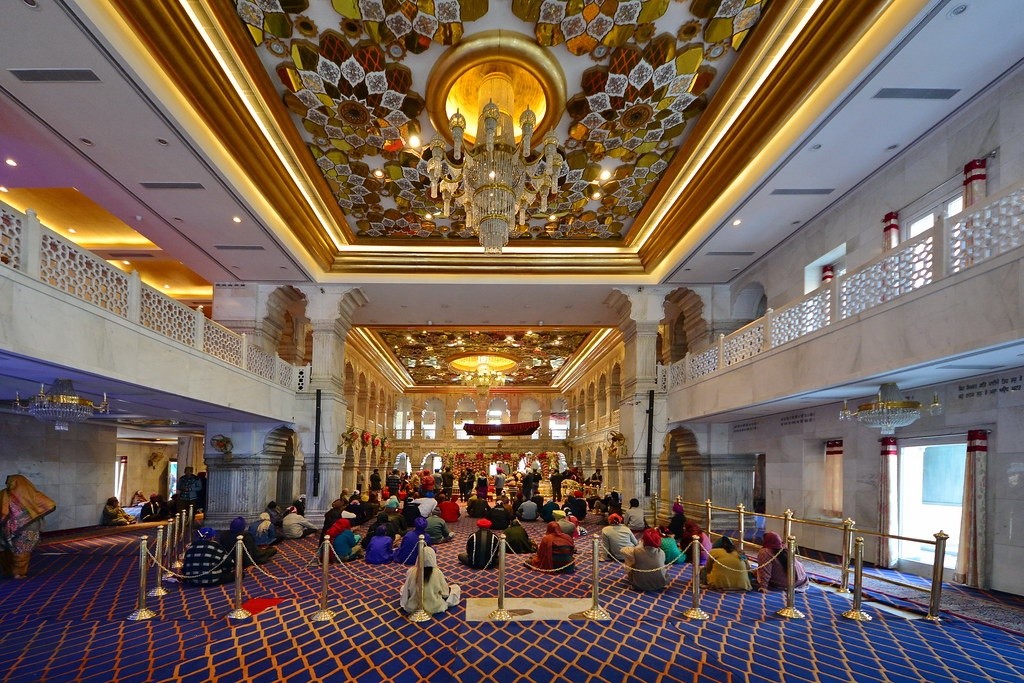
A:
607,430,627,455
210,434,233,454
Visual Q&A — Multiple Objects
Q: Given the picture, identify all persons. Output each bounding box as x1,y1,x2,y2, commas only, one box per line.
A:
698,536,753,591
458,518,499,570
599,513,637,562
504,518,537,553
620,528,671,591
104,497,136,527
681,520,713,566
751,531,810,593
220,516,277,570
523,507,579,575
183,528,245,587
264,494,319,539
318,467,460,563
654,525,686,563
667,503,686,541
248,512,285,546
623,498,650,530
0,474,56,579
400,546,461,613
132,467,207,521
458,467,622,530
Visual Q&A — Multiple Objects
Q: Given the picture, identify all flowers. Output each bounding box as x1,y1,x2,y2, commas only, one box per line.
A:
371,434,379,449
381,437,390,451
360,430,371,447
346,428,359,445
443,451,560,469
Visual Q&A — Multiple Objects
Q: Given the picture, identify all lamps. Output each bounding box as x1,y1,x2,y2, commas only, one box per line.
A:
838,382,943,435
459,356,506,402
426,71,563,257
10,378,110,432
